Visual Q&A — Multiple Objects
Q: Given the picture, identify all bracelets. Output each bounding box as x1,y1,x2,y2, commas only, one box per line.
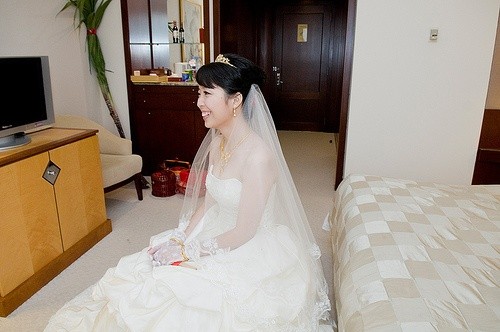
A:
181,247,189,261
170,237,184,246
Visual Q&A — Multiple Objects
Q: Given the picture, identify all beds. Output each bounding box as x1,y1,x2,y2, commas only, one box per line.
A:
321,174,500,332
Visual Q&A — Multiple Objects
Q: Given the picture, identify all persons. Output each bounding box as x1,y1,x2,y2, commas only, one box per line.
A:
43,54,336,332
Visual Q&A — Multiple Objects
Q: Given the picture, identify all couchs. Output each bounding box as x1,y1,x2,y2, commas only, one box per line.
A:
53,115,144,200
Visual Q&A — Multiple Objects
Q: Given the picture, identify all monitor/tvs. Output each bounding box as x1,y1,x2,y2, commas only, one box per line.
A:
0,56,55,149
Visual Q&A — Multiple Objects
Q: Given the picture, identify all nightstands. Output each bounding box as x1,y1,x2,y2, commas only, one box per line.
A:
469,109,500,185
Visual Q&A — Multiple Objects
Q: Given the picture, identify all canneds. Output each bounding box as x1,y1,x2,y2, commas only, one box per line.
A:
182,72,189,82
185,70,192,82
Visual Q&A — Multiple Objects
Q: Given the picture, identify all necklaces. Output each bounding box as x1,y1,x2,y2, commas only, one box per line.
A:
218,134,248,175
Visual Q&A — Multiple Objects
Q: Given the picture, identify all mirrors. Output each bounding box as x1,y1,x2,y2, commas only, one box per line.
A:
119,0,211,86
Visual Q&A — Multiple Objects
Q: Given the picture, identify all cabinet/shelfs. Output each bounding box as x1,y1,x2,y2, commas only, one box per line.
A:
136,77,211,176
0,125,113,319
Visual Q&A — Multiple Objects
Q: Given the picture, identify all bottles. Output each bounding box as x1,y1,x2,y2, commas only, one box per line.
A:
172,20,178,43
179,22,185,43
